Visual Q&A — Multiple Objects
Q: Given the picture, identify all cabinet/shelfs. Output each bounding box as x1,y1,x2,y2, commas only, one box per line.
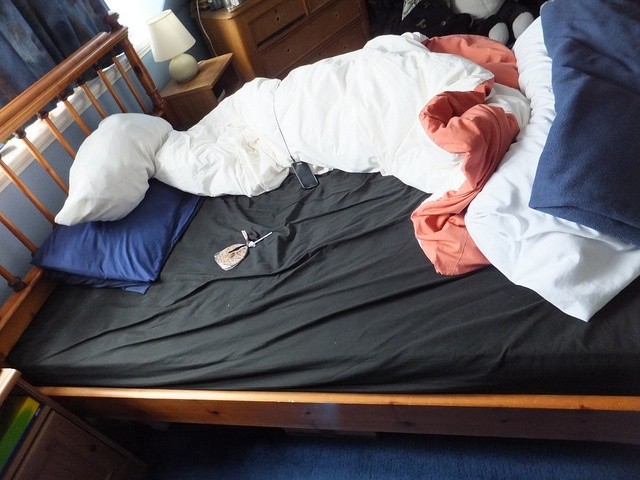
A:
198,2,372,82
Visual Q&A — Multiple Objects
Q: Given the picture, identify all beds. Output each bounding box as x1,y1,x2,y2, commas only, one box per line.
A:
1,11,640,444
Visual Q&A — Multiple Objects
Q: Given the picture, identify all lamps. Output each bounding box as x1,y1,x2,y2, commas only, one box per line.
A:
145,9,199,83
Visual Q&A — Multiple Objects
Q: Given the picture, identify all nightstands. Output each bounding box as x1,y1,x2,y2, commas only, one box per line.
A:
1,366,149,476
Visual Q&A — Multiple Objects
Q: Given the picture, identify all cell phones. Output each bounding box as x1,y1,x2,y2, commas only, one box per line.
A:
291,161,319,189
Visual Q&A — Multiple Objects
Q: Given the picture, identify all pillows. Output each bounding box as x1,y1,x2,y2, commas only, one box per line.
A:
54,114,173,227
29,179,203,294
